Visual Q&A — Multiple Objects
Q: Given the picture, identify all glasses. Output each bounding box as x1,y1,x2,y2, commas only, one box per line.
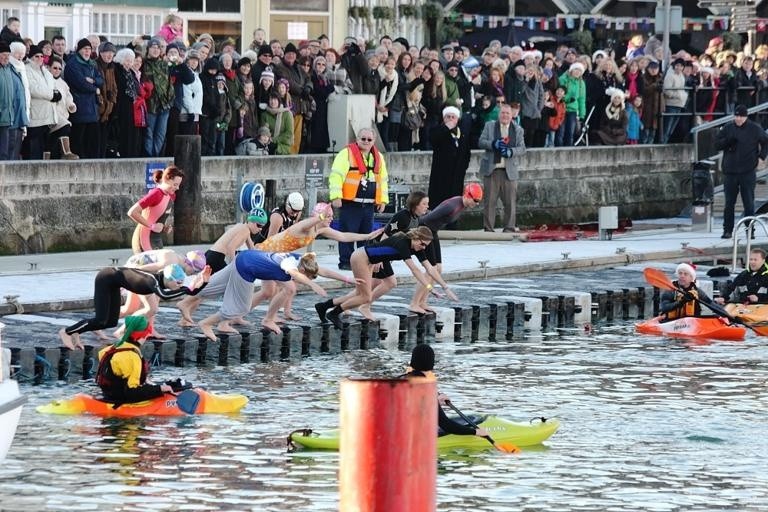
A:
360,137,373,142
420,240,428,247
469,193,480,204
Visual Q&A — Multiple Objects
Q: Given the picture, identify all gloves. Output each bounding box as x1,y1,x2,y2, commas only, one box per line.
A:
492,137,512,158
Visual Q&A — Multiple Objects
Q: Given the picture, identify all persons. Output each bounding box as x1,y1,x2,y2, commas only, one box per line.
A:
178,252,366,341
661,263,730,325
178,208,266,326
398,344,491,432
233,202,386,334
409,183,483,313
715,106,768,239
330,128,388,269
58,265,211,349
255,193,303,304
0,16,764,159
344,192,429,322
94,249,206,340
715,248,766,304
430,106,473,229
95,315,192,403
127,166,183,255
315,226,458,330
478,106,525,232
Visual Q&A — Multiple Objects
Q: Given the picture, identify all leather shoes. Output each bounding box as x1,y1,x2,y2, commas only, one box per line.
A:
721,231,732,239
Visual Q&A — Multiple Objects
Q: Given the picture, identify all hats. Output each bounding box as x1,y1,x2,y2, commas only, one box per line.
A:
160,193,333,283
257,127,271,137
113,315,153,349
447,40,684,110
676,262,697,282
464,184,482,200
734,105,748,116
410,344,435,372
29,35,310,105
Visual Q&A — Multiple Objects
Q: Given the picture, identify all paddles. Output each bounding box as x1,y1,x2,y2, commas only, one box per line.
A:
436,389,522,453
644,267,768,337
144,380,200,414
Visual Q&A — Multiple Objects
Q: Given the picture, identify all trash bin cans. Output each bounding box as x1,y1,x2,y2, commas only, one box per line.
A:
690,200,712,235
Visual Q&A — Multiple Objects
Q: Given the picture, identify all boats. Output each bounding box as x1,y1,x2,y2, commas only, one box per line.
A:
34,388,250,423
631,298,768,340
287,411,559,460
